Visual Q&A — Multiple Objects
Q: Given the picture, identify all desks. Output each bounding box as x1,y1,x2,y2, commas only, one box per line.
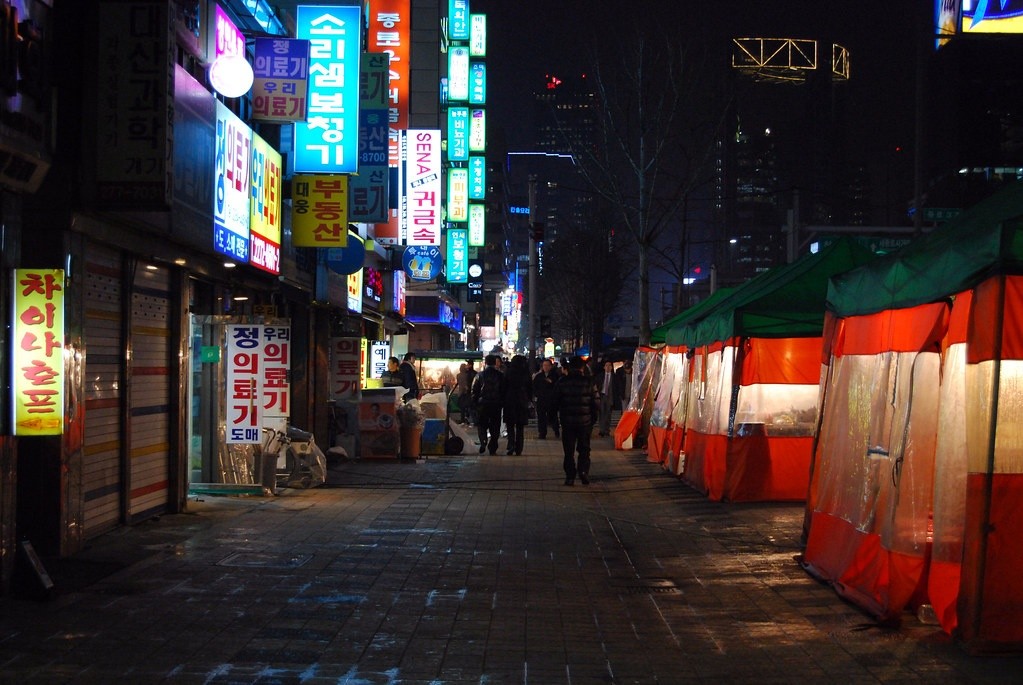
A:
765,424,815,436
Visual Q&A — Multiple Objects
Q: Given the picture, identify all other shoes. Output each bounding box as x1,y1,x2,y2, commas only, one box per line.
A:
479,440,487,453
605,433,609,436
516,451,521,456
599,433,604,437
565,476,574,486
579,472,590,485
507,446,515,456
538,435,546,439
555,430,559,437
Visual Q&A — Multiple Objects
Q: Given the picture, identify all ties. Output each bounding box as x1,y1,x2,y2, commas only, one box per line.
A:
605,374,609,396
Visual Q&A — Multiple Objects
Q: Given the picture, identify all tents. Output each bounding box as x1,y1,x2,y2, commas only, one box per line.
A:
614,176,1022,657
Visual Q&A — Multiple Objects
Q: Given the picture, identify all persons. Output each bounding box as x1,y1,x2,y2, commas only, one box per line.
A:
553,357,599,487
499,356,533,456
399,352,419,404
440,355,633,441
381,357,404,387
470,354,505,456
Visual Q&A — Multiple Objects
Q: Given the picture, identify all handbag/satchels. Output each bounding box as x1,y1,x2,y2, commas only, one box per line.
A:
468,403,485,425
527,402,534,418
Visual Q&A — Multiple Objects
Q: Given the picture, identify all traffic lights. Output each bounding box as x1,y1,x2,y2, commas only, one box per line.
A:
533,218,547,245
